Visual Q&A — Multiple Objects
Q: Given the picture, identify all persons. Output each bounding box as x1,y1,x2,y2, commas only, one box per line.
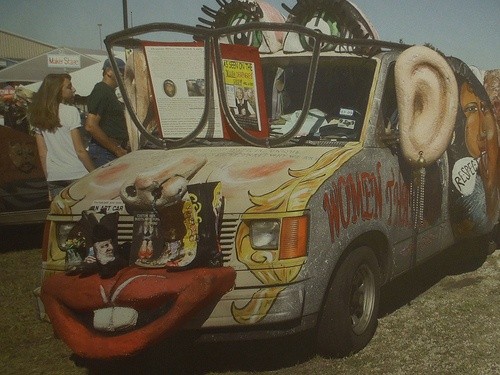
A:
165,81,175,97
235,87,250,117
24,72,96,201
92,226,116,264
84,57,131,168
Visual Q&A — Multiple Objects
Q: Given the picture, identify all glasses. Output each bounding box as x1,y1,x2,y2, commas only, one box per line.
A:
110,68,125,73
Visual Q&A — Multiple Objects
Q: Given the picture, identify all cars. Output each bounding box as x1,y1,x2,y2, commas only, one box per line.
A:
33,0,500,361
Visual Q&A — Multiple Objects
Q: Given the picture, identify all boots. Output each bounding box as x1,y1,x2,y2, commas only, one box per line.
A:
63,212,131,278
134,174,224,271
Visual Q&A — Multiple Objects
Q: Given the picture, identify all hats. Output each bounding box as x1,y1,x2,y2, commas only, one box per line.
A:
102,57,125,69
